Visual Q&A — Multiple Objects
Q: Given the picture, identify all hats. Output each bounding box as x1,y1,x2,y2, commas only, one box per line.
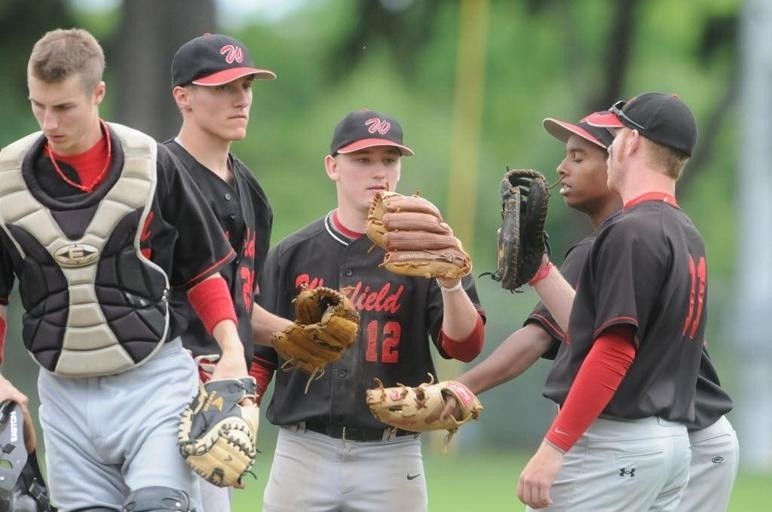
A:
170,32,278,92
330,109,414,159
541,91,698,158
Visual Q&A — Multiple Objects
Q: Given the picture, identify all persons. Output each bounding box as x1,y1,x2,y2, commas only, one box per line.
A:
496,90,712,512
145,32,361,512
1,27,261,511
250,108,486,512
368,104,742,512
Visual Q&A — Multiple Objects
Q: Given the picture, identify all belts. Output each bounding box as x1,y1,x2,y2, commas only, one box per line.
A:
598,406,685,428
287,417,421,442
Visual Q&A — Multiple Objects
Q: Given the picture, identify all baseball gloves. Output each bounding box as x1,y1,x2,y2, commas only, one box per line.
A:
366,182,473,279
270,282,361,394
366,373,484,454
177,376,263,486
478,166,568,293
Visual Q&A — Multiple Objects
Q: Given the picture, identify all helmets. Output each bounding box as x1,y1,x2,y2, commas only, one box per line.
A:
0,399,50,512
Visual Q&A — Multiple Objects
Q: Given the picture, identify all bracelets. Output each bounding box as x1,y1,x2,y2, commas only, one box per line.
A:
434,279,463,293
